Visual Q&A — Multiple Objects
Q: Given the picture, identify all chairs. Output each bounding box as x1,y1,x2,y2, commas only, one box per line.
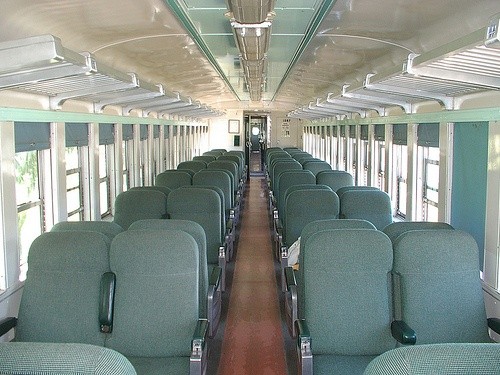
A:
265,146,500,375
0,148,247,375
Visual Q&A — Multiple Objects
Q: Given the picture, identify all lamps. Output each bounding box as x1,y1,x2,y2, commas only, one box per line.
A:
226,0,276,104
